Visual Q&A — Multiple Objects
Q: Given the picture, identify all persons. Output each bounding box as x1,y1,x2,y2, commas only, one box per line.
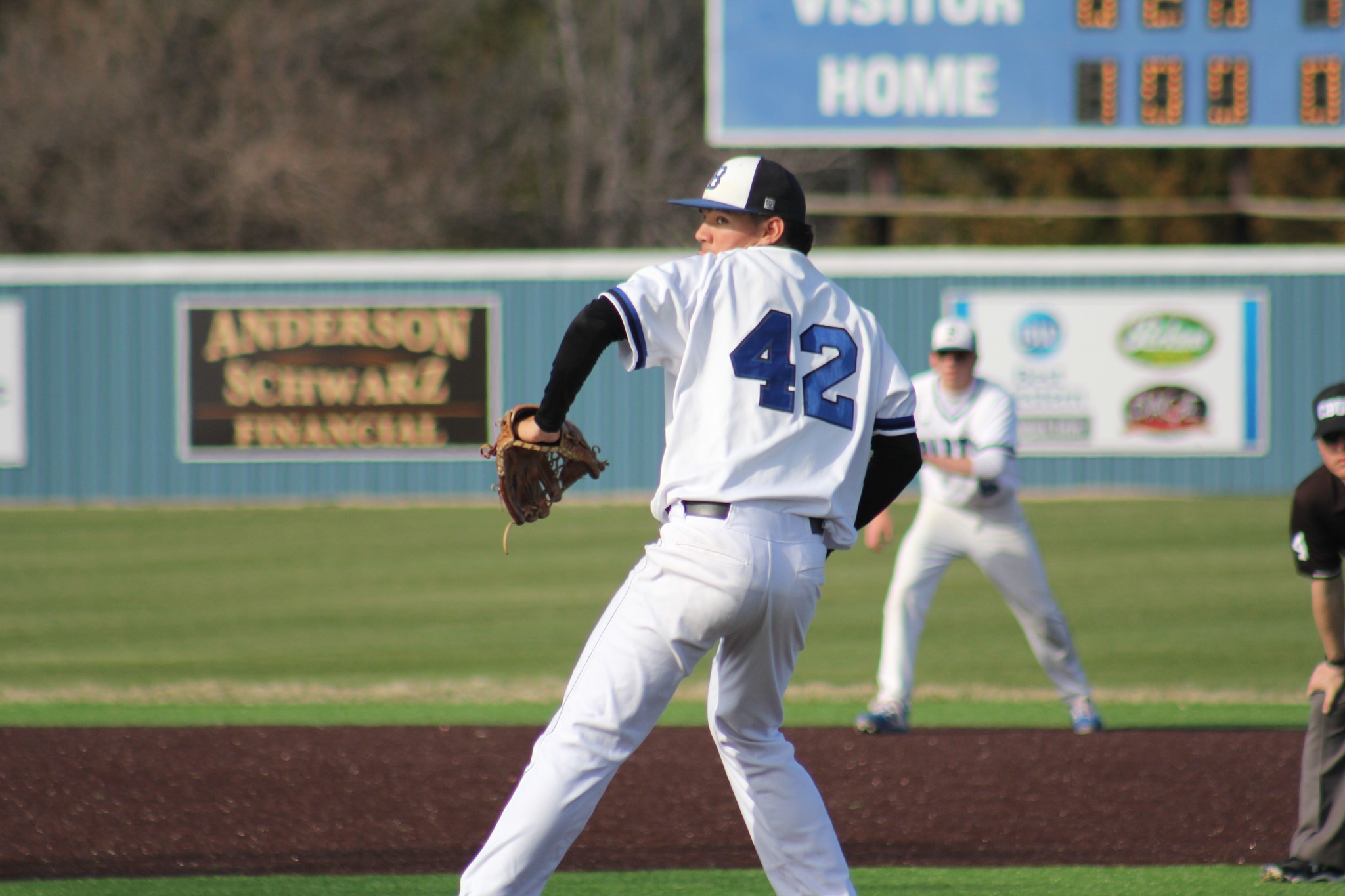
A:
1260,381,1345,887
456,156,922,896
853,314,1104,737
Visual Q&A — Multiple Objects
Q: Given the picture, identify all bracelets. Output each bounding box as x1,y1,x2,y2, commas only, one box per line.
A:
1323,655,1345,667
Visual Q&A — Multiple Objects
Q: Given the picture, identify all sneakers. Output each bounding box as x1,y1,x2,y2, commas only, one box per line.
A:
855,699,910,733
1260,857,1345,884
1069,690,1102,734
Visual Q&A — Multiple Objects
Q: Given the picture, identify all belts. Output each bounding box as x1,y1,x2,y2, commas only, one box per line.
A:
665,500,823,535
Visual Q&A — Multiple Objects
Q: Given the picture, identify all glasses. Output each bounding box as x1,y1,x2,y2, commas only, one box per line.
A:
1322,431,1345,443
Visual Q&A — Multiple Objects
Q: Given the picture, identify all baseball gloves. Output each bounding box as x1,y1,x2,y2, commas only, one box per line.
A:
494,404,607,526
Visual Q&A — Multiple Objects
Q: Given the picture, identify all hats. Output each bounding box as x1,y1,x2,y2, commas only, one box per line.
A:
668,156,806,222
1310,383,1345,441
931,317,976,352
